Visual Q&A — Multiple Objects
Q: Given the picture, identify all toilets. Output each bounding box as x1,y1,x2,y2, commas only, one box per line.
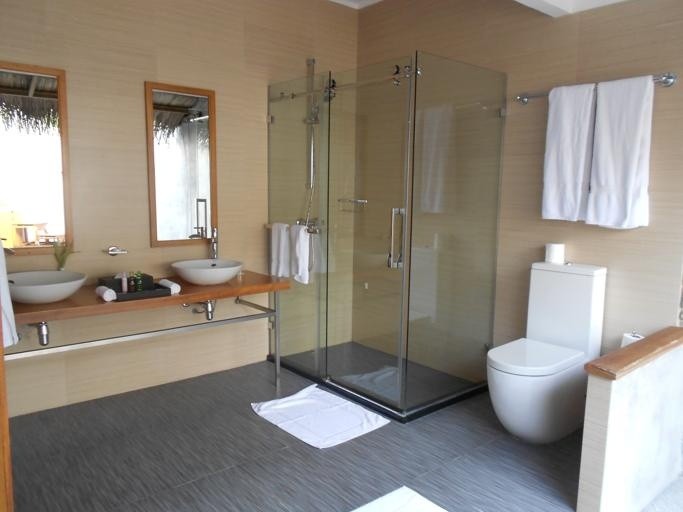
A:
487,262,608,445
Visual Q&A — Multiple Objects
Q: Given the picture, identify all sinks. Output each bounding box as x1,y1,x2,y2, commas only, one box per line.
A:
6,271,87,304
170,259,243,286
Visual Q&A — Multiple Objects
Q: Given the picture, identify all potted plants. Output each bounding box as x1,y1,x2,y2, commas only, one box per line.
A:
52,235,71,271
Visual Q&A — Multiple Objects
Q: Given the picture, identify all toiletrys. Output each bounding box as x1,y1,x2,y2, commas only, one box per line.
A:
122,270,143,294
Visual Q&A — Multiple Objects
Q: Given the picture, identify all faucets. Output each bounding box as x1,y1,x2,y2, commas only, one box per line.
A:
0,237,18,257
189,227,203,238
208,228,217,259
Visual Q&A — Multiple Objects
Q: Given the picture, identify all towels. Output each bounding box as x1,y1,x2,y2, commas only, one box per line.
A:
0,238,19,348
421,103,453,213
541,75,596,229
290,224,310,285
585,75,654,230
250,383,392,450
271,223,290,278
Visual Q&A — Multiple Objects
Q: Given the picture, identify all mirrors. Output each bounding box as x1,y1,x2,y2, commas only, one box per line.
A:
0,61,73,256
144,81,218,248
